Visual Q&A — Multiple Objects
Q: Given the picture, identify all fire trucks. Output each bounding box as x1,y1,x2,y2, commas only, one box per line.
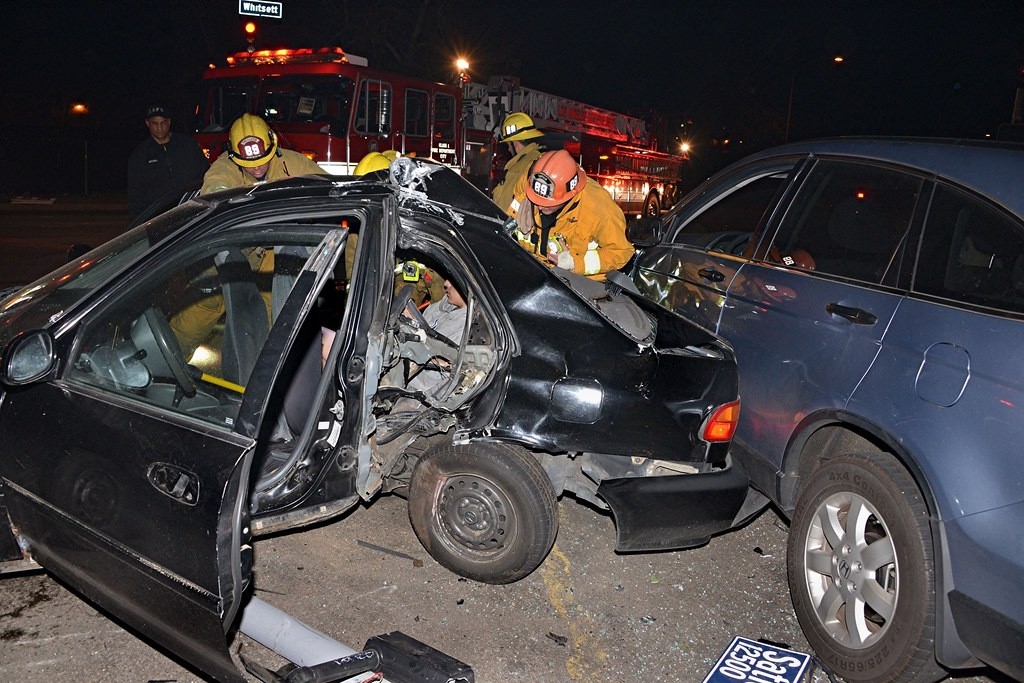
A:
194,49,687,217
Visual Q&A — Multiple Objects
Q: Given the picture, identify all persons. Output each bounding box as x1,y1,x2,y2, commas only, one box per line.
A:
118,102,211,221
492,113,544,211
169,113,327,364
345,149,447,308
375,280,468,391
517,150,634,280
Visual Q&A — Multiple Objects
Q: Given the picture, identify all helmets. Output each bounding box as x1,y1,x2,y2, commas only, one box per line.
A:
524,149,587,208
497,112,545,144
227,112,278,168
352,150,401,177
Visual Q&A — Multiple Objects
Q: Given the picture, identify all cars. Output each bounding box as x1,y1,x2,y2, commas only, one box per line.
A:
0,155,751,683
620,132,1024,527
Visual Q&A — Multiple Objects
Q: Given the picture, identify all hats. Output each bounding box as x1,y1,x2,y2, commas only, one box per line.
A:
143,98,178,117
